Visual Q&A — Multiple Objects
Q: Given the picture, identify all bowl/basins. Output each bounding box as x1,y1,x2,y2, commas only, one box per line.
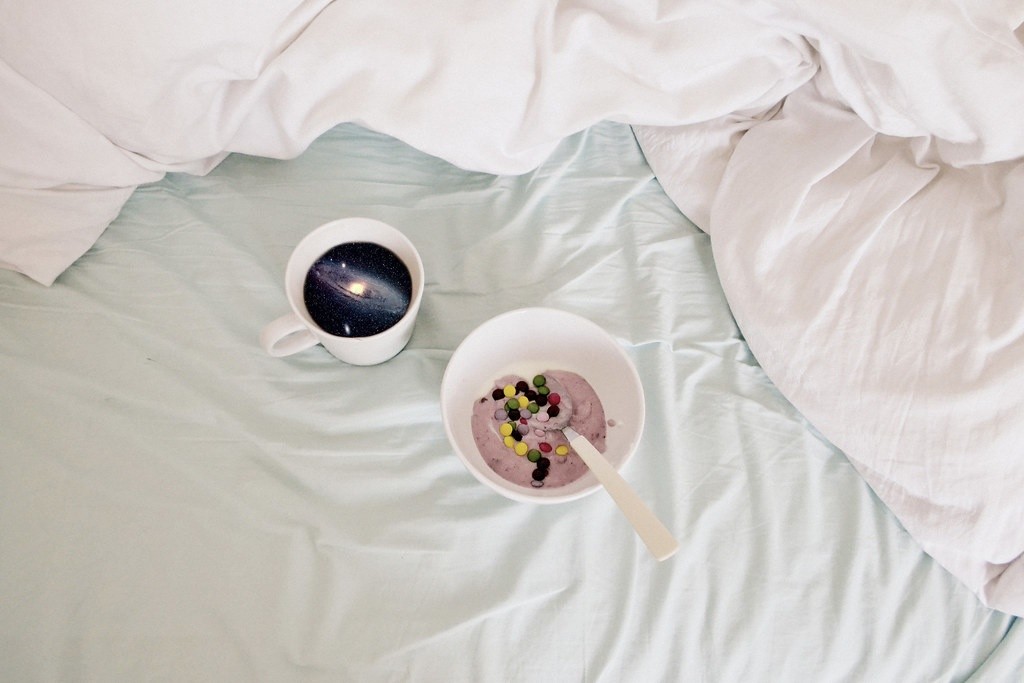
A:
442,307,646,505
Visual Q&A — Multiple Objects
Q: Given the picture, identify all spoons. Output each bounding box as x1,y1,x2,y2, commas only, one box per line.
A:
520,374,681,562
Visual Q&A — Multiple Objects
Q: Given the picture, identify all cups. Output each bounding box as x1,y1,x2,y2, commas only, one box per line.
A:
259,217,424,367
